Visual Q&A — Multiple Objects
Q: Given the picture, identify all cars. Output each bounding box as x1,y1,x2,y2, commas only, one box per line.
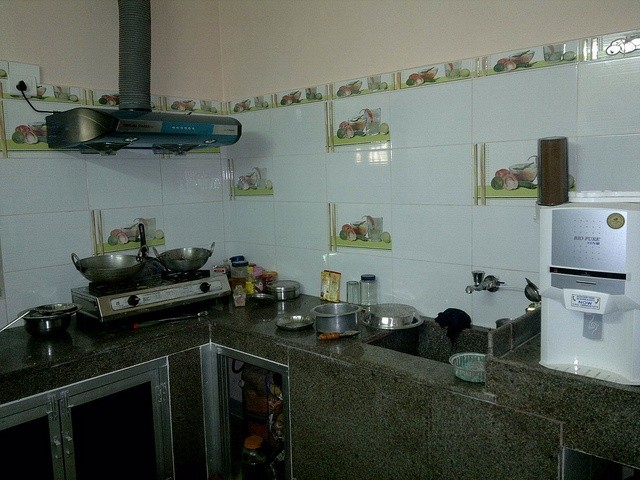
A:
367,75,382,92
51,86,71,100
198,100,211,113
253,96,264,109
305,87,318,99
365,107,381,137
542,44,564,66
138,218,156,241
367,216,383,242
254,168,267,190
346,280,360,305
445,60,462,80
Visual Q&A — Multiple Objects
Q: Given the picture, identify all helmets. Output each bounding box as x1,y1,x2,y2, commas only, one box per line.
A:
247,292,273,303
359,303,427,332
278,316,314,332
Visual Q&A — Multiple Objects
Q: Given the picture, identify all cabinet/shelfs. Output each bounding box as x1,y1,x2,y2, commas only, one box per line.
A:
242,436,265,479
361,274,376,307
223,258,230,278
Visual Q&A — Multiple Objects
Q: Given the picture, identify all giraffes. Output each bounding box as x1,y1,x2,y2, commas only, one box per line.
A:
71,270,232,323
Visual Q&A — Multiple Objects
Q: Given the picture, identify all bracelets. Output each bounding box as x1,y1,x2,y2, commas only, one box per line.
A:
311,302,362,334
449,352,488,383
18,303,82,335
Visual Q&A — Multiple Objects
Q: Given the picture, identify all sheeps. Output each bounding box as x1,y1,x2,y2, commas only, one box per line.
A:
463,264,503,299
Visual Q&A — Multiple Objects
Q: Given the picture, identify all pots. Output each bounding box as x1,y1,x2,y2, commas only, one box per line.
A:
154,242,216,271
71,244,150,284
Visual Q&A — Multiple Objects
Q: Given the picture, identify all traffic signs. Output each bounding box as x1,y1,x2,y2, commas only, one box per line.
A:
8,74,37,98
